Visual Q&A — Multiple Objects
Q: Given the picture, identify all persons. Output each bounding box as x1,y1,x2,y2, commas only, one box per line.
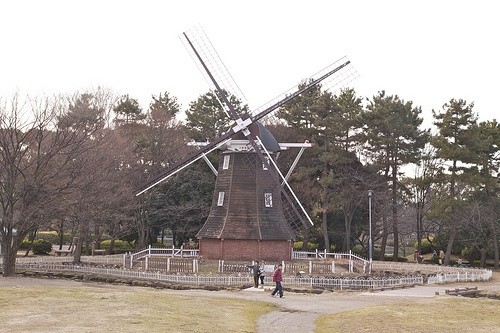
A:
269,261,289,298
437,247,445,267
414,249,420,265
456,256,463,267
246,260,260,289
258,260,266,289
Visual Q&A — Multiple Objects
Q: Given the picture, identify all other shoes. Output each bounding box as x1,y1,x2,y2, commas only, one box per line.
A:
254,286,257,288
270,294,275,298
280,296,285,298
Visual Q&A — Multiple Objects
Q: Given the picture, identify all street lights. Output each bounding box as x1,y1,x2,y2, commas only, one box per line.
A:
366,189,375,274
